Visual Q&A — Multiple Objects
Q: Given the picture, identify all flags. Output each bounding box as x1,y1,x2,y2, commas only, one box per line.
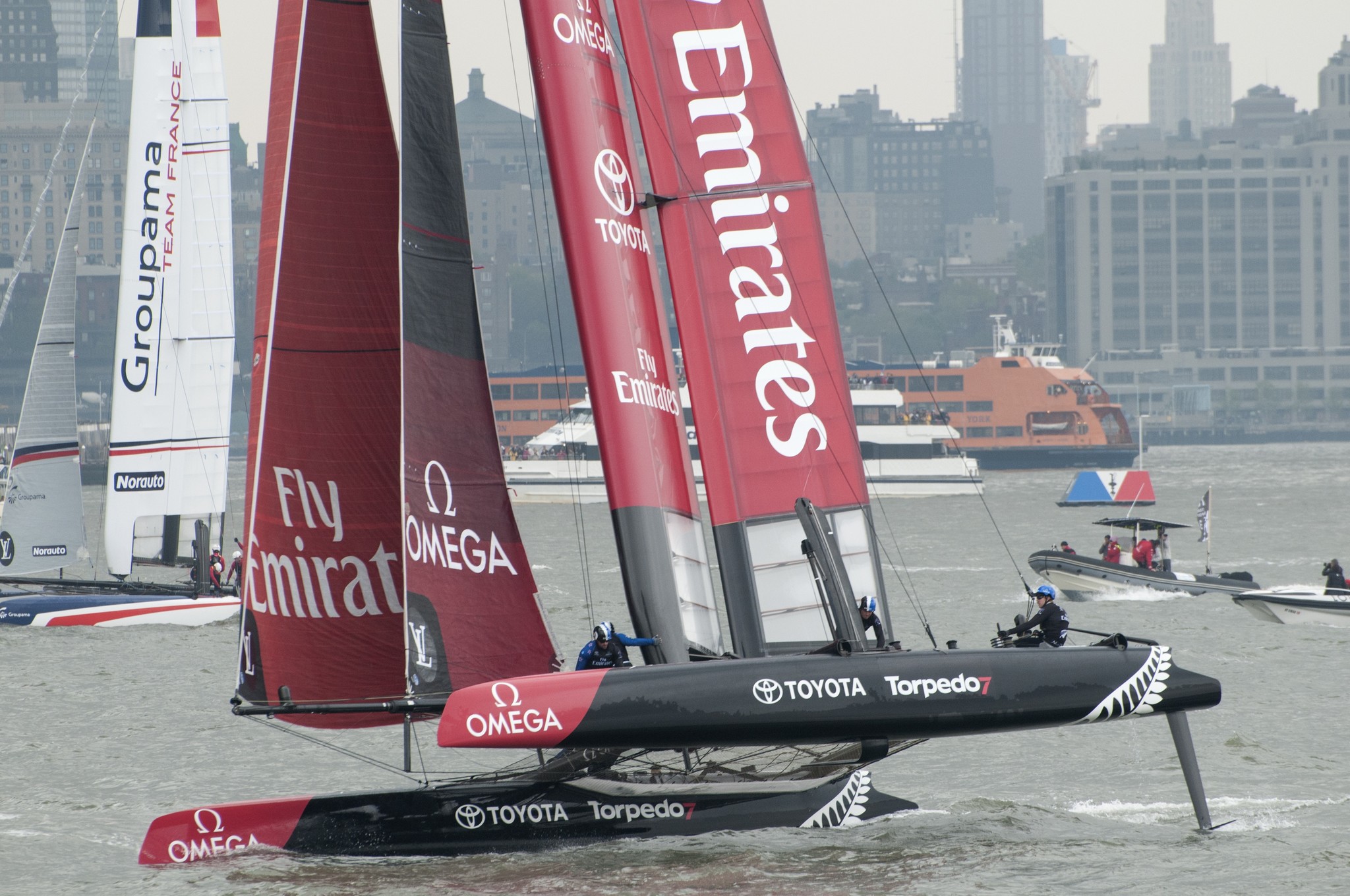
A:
1194,489,1210,541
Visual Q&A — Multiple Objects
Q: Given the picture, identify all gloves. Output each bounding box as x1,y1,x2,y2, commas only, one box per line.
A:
234,537,238,542
997,631,1007,639
220,588,224,594
226,581,229,586
652,635,661,646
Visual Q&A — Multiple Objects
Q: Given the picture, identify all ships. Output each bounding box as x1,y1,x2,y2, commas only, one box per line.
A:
485,313,1150,469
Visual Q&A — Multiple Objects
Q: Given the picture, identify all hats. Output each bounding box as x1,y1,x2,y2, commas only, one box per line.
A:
1109,535,1118,544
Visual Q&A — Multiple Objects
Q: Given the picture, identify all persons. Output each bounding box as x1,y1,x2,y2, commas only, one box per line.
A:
1129,533,1172,574
497,441,587,460
1098,534,1114,560
833,594,886,653
232,537,243,551
1103,536,1121,565
1061,541,1077,555
208,544,226,591
997,584,1069,649
189,561,222,595
225,550,243,597
1322,557,1349,597
600,620,663,668
576,625,627,671
846,370,954,427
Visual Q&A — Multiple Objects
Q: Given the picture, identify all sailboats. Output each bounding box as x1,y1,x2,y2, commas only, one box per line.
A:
136,0,1237,866
2,0,257,630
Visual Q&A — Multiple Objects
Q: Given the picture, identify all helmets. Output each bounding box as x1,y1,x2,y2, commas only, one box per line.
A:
233,551,241,559
214,562,222,573
601,621,615,634
859,596,876,612
212,544,220,551
593,624,612,641
1038,585,1056,600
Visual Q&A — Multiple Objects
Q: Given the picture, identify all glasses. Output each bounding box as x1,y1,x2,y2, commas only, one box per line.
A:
599,641,609,643
1037,595,1049,599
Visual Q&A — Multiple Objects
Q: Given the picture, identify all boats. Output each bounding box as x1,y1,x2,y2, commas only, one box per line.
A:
501,345,988,502
1230,589,1350,630
1055,464,1156,509
1024,510,1264,604
